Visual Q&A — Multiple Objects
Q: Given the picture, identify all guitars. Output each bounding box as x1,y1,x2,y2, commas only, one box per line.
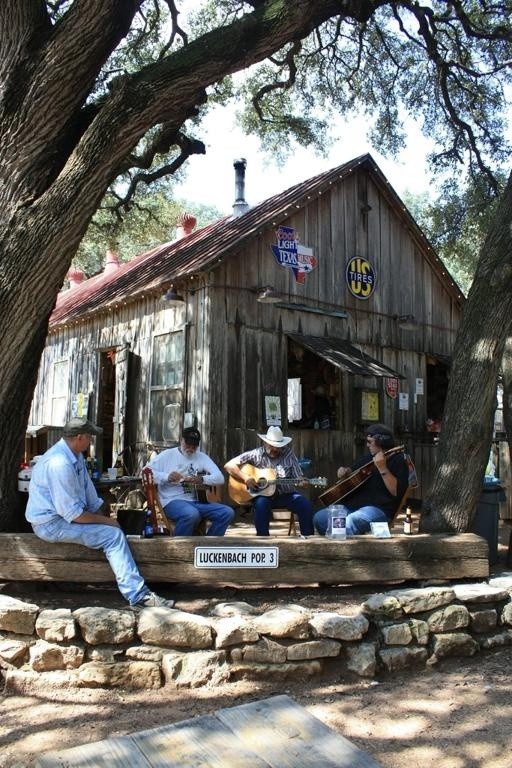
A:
179,472,222,504
318,445,404,507
229,463,328,505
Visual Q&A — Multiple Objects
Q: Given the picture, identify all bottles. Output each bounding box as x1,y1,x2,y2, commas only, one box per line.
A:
144,505,153,538
326,504,346,540
86,456,100,481
111,453,123,478
403,504,413,536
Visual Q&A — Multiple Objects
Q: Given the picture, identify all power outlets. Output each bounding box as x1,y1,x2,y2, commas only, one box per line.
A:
251,283,283,304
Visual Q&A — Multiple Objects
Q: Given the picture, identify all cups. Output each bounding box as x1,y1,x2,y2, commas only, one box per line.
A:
108,468,117,481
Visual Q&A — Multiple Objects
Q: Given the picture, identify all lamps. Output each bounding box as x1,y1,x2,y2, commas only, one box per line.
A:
159,287,196,306
393,313,422,331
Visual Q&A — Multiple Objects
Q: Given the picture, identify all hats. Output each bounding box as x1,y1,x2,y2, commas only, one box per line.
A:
256,426,293,448
182,427,201,445
62,417,104,436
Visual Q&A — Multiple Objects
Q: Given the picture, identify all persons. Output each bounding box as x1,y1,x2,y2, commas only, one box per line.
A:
313,423,409,535
225,425,314,536
25,420,174,610
141,428,234,536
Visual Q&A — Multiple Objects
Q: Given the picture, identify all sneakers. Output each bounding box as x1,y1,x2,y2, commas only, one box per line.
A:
129,591,176,612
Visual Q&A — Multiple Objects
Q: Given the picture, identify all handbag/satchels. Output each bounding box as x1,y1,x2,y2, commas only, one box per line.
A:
117,509,149,535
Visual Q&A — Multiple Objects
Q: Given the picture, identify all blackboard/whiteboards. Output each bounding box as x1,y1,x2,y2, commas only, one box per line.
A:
72,394,90,419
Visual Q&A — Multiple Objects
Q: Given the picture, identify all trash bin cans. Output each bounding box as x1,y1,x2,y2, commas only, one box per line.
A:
474,483,506,565
